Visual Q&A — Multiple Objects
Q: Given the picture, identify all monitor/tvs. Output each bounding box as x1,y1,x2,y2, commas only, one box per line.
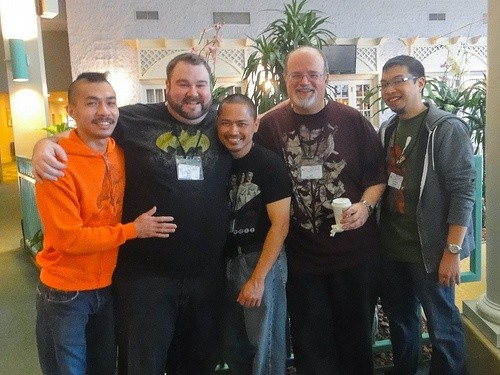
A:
316,44,356,75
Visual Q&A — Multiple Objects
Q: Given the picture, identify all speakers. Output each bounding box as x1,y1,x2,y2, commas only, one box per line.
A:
40,0,59,18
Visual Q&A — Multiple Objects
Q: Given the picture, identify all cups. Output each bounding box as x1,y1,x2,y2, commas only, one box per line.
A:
332,197,352,226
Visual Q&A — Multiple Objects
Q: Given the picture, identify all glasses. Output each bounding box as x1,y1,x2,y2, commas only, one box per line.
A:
289,73,325,82
376,77,419,90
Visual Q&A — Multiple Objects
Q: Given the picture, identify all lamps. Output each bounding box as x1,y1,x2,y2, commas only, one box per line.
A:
8,39,31,83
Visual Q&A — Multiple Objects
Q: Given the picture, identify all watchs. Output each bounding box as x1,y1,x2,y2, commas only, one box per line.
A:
446,242,463,254
360,199,373,216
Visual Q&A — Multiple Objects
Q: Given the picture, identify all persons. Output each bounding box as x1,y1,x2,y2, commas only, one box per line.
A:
379,55,475,375
32,53,294,375
252,45,389,375
